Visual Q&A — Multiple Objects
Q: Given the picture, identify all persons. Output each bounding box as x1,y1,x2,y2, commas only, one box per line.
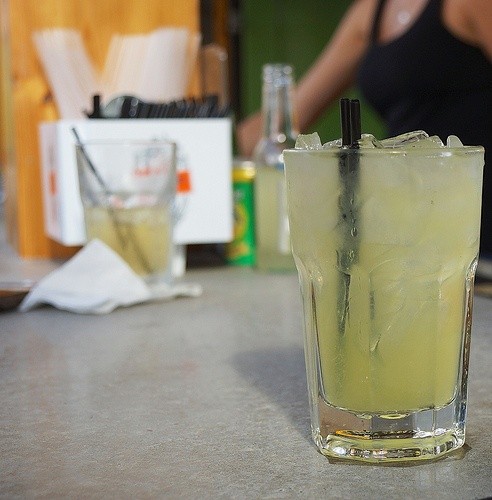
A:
235,1,492,291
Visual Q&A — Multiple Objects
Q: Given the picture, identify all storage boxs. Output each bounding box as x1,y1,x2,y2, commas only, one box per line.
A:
37,118,232,247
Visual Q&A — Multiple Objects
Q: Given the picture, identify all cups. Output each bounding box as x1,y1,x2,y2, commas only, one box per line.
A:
76,142,176,286
281,148,484,463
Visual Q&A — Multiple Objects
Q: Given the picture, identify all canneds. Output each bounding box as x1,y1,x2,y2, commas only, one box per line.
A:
210,158,255,265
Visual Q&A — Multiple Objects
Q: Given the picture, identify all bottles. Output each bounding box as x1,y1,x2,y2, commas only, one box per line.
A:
253,64,300,270
